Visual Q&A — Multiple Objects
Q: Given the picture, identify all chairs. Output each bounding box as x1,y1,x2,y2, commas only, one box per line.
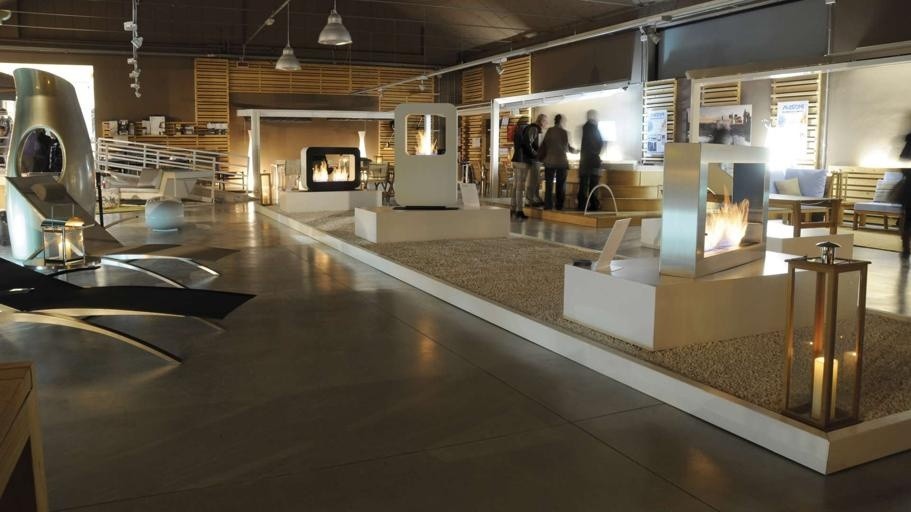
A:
853,172,905,230
769,168,834,225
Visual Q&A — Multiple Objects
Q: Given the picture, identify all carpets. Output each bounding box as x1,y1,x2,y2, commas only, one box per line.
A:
836,226,905,252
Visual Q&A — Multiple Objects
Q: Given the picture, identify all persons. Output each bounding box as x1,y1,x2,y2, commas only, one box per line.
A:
576,109,602,210
509,116,535,220
537,114,579,210
525,113,549,207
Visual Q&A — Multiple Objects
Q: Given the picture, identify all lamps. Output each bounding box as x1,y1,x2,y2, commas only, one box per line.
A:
274,1,354,73
123,19,145,99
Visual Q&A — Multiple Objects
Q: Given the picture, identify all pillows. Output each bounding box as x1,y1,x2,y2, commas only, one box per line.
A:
776,177,802,196
873,180,901,203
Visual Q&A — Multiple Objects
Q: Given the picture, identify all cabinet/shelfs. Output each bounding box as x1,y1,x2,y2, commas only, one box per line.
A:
781,255,873,433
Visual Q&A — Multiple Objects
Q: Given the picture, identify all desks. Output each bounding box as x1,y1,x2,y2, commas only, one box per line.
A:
769,197,844,238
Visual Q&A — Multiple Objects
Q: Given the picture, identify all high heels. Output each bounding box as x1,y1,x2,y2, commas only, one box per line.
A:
510,209,529,219
